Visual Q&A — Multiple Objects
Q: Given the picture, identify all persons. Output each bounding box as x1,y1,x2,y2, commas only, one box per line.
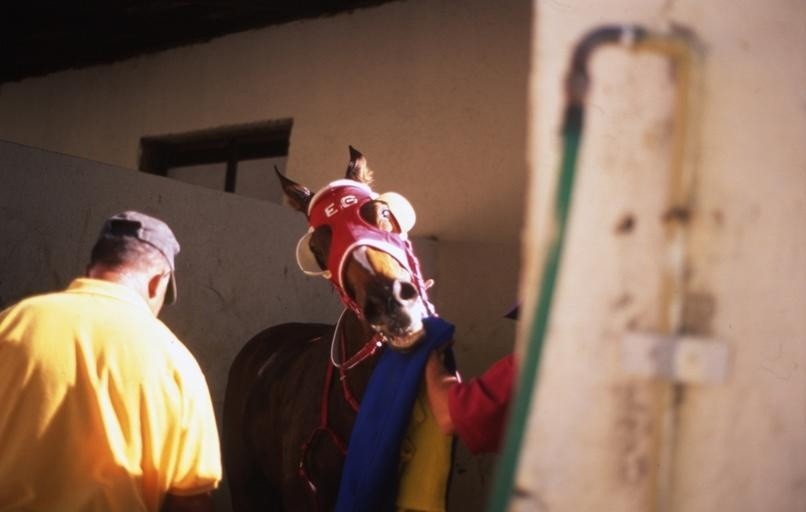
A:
426,304,519,510
0,209,223,511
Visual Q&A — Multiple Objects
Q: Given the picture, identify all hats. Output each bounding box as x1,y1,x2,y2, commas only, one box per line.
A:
96,211,180,307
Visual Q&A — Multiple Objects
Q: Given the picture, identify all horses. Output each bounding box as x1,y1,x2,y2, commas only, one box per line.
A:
220,145,458,512
